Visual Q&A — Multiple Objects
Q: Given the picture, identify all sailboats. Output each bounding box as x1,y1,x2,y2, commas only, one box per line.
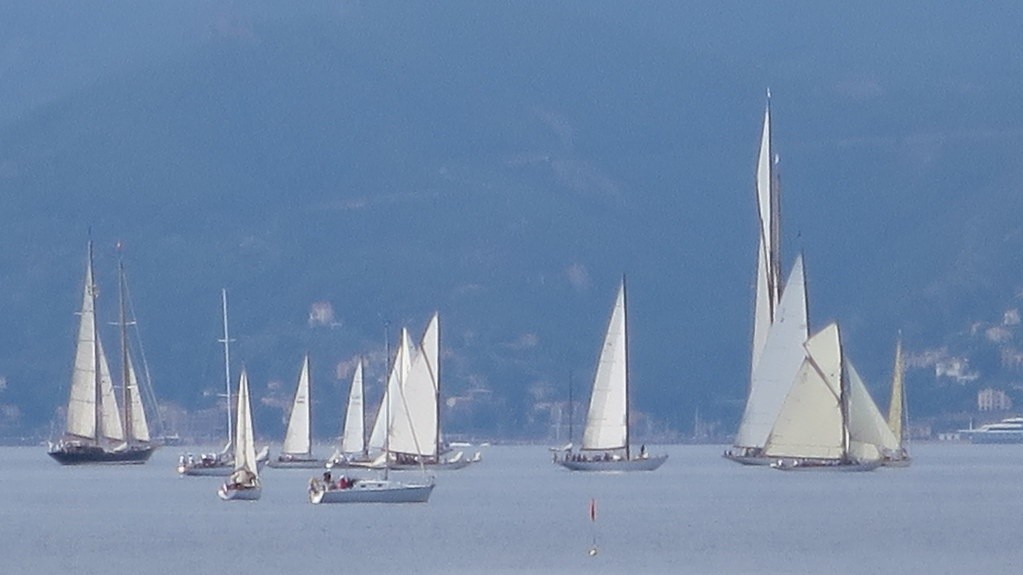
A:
722,82,914,475
552,268,668,473
45,224,180,469
325,309,480,471
175,286,270,477
307,324,437,506
216,364,262,502
267,354,327,469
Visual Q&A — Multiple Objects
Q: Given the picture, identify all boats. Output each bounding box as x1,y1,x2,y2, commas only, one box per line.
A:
966,414,1023,445
